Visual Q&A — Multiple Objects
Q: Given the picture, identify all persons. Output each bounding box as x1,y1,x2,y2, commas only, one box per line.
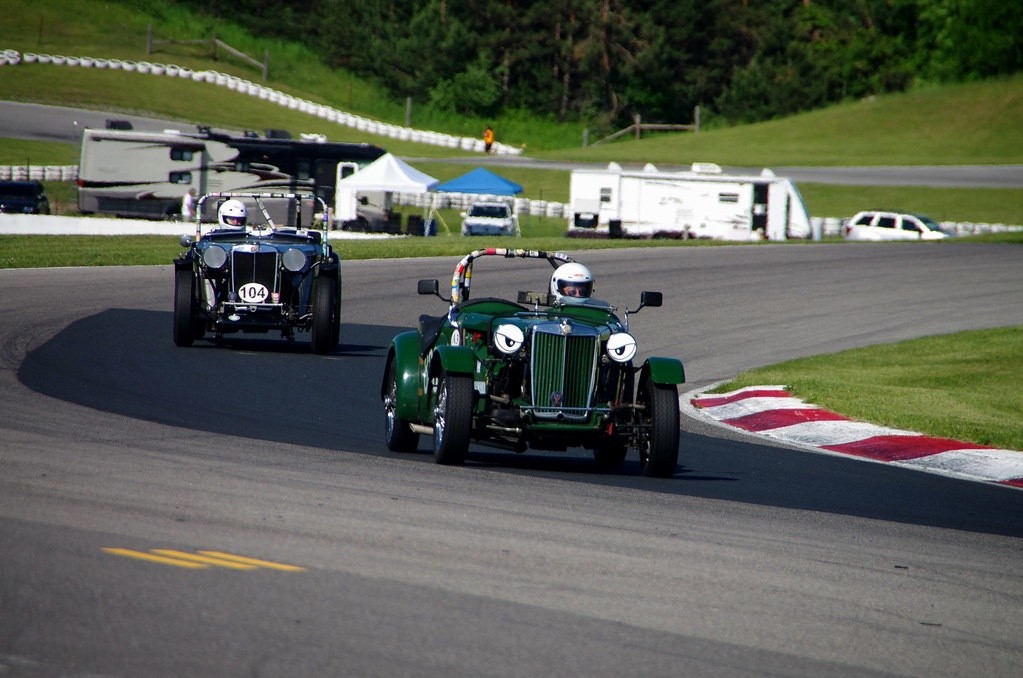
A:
483,123,496,153
550,262,597,302
181,187,197,219
211,200,247,232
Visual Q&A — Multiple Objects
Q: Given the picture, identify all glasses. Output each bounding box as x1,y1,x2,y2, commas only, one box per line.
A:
567,288,586,297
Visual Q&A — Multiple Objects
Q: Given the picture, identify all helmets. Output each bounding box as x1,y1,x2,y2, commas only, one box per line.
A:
550,262,594,304
218,199,247,231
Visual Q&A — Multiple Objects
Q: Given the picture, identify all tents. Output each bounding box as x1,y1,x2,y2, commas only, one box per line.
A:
331,153,440,231
426,166,522,238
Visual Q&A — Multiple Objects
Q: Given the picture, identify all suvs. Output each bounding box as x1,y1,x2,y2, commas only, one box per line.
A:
844,208,955,242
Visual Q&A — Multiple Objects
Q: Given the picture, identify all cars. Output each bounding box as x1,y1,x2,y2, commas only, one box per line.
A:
378,245,685,480
460,201,519,239
0,179,51,215
172,191,342,357
